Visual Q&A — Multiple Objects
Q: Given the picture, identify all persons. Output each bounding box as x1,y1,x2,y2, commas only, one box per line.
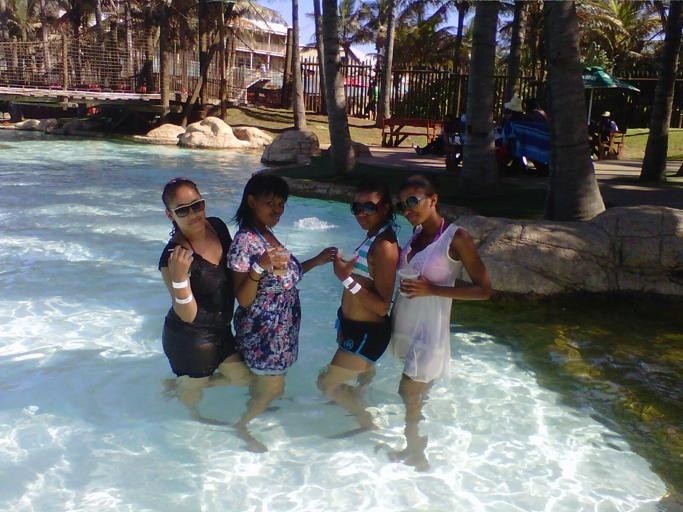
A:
390,173,493,475
312,181,398,444
226,174,337,456
411,93,619,176
155,177,249,430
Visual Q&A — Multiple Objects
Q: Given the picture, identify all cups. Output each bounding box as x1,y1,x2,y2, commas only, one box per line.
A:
272,249,292,275
395,267,420,298
338,246,359,263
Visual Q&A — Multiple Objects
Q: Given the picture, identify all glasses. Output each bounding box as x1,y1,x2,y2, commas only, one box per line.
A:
351,202,377,216
396,197,427,212
170,199,204,217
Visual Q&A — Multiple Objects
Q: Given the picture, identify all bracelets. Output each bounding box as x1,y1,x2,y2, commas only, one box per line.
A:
348,282,361,294
247,271,260,283
251,261,264,277
170,278,188,289
340,276,354,287
174,293,192,304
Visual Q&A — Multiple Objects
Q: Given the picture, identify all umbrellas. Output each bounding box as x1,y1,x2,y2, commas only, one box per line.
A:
580,65,643,126
